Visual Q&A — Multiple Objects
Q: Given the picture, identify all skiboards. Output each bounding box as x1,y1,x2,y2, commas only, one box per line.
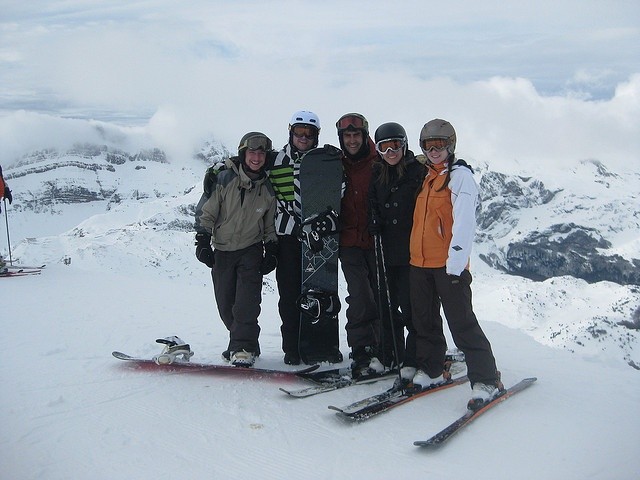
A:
336,374,537,447
280,347,468,412
0,264,47,278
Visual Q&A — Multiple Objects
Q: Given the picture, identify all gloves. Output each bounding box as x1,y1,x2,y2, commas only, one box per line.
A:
260,245,279,275
367,213,384,238
203,170,218,198
448,269,472,303
324,144,343,160
194,231,215,268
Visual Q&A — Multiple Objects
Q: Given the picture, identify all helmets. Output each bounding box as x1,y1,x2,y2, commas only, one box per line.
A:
239,132,272,162
374,123,408,160
288,110,320,150
336,112,370,159
420,119,456,161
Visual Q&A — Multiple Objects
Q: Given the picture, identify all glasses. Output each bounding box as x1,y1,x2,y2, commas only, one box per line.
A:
336,115,367,130
288,124,321,140
238,135,272,154
419,133,455,153
374,135,407,155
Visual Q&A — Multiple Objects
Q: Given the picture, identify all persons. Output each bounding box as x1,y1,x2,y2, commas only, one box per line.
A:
0,169,14,275
193,131,280,367
336,113,376,380
367,121,428,389
202,109,348,366
409,118,505,411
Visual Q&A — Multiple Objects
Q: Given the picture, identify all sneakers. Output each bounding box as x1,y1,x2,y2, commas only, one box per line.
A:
368,348,405,379
220,344,261,361
396,364,416,388
468,381,505,412
411,369,447,391
230,348,257,369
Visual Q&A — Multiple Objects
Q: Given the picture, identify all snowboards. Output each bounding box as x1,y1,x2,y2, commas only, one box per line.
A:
293,364,365,385
112,350,319,374
299,148,346,365
